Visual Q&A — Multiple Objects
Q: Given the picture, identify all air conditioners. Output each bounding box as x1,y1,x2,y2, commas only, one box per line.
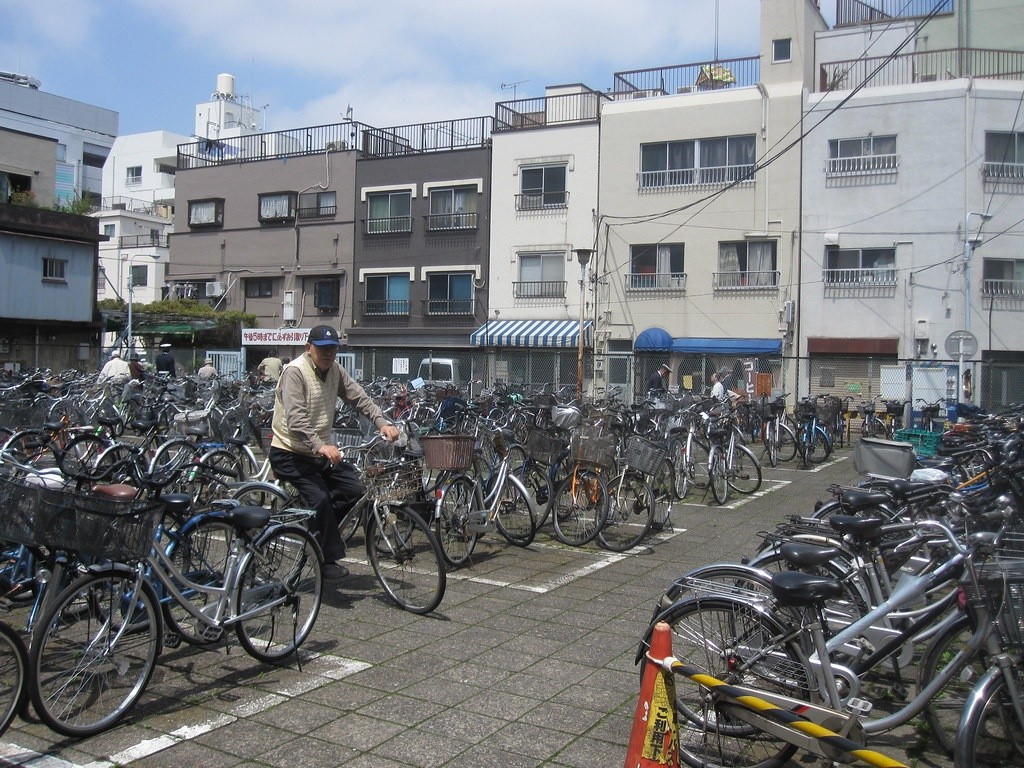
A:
205,282,225,296
326,140,345,152
632,88,666,99
675,86,698,94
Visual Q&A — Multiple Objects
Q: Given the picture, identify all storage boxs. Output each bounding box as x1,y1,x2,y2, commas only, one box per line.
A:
852,436,918,479
897,428,945,459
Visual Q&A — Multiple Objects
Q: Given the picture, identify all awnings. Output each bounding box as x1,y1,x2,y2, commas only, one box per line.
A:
668,338,781,355
634,327,673,352
469,320,594,349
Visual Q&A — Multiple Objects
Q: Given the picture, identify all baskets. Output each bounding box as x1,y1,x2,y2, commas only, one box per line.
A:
0,474,162,561
362,454,424,500
962,559,1023,641
3,381,275,443
331,398,945,474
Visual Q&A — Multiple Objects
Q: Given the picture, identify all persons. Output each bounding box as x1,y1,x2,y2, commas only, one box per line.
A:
257,350,283,387
709,373,724,400
268,323,398,580
155,343,176,377
97,350,132,382
647,364,674,393
127,354,144,382
198,358,218,378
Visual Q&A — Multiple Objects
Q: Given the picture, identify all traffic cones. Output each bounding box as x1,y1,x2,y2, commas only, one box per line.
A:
623,615,681,768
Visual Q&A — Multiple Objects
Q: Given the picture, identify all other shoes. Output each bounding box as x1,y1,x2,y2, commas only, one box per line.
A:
314,561,349,577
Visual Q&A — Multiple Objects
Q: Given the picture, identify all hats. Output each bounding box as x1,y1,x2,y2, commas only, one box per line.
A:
308,325,341,347
129,354,140,360
662,363,673,374
205,358,212,365
111,351,120,357
161,344,171,352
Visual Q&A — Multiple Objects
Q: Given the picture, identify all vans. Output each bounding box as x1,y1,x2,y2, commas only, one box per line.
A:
414,359,469,394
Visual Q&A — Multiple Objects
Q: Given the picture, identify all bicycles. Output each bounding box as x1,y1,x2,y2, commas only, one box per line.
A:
1,366,1024,768
